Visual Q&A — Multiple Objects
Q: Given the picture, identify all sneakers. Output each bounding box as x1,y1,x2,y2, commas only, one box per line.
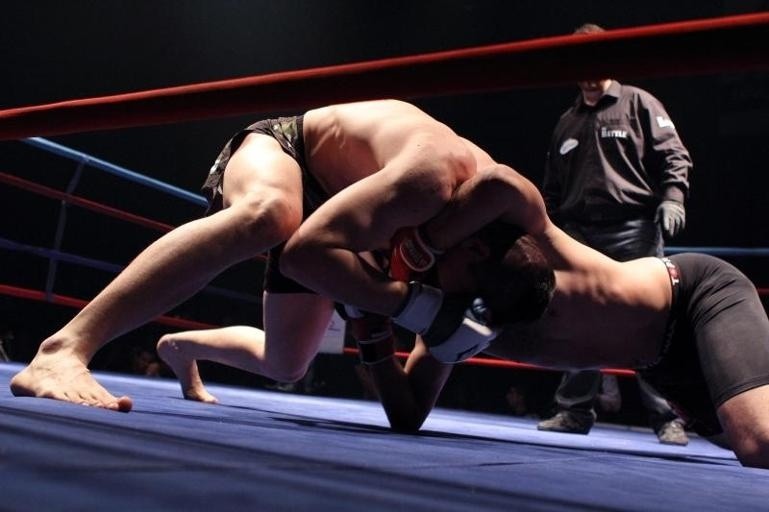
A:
537,408,597,436
656,419,688,447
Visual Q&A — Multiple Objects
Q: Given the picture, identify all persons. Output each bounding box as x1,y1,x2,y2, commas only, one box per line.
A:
10,94,555,412
341,164,769,468
504,378,539,420
536,20,691,443
595,370,625,421
131,341,164,377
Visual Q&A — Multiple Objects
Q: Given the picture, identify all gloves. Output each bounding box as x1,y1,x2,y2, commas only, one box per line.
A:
654,197,686,237
395,280,502,366
388,226,449,278
334,300,398,367
